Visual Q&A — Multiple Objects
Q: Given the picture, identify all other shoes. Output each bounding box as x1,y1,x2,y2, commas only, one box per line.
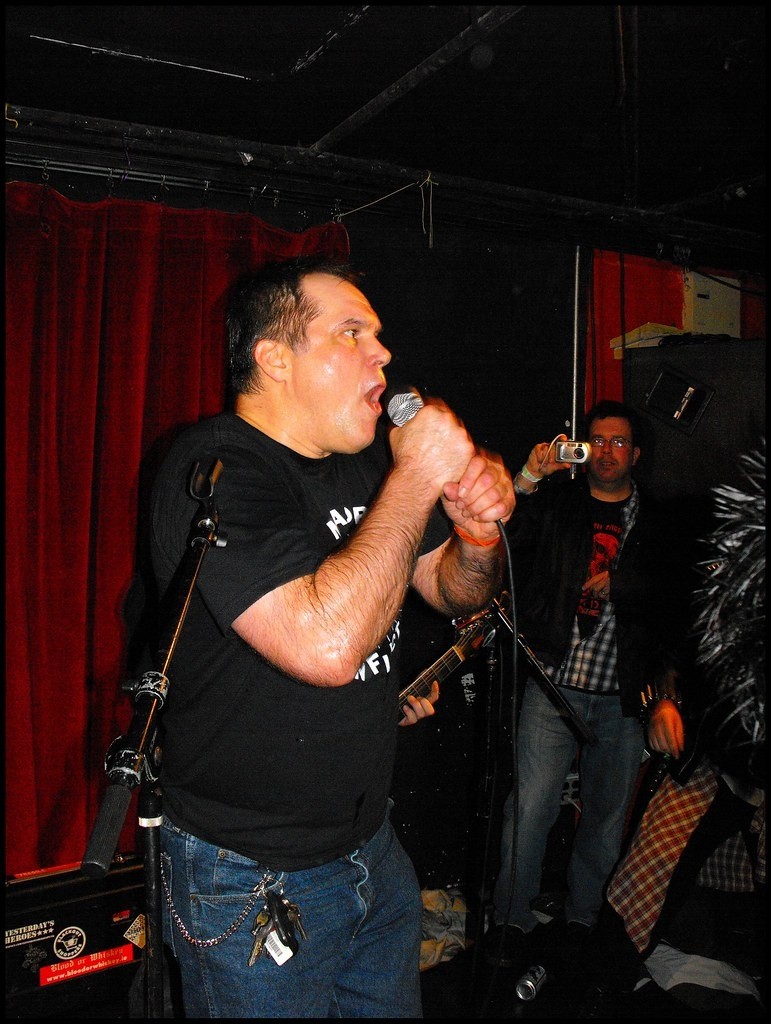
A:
564,923,609,965
488,925,525,962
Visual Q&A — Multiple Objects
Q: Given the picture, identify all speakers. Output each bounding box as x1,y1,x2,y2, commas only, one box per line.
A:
2,852,186,1020
620,337,770,715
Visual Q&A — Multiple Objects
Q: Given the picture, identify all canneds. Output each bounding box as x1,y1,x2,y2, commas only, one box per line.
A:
515,965,547,1001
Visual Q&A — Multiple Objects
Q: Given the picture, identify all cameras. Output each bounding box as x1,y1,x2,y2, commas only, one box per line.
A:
555,439,593,463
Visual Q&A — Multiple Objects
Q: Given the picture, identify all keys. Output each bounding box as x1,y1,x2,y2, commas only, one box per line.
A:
246,889,307,969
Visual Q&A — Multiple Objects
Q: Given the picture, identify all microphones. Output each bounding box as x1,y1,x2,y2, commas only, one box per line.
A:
386,394,423,428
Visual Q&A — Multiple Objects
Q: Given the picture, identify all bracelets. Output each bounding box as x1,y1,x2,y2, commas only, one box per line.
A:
637,682,681,724
453,525,501,546
511,475,539,495
521,465,543,483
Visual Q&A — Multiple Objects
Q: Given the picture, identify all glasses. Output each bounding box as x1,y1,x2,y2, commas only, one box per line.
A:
588,434,632,448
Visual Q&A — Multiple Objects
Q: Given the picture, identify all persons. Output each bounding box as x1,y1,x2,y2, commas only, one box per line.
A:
119,252,518,1024
494,399,770,963
